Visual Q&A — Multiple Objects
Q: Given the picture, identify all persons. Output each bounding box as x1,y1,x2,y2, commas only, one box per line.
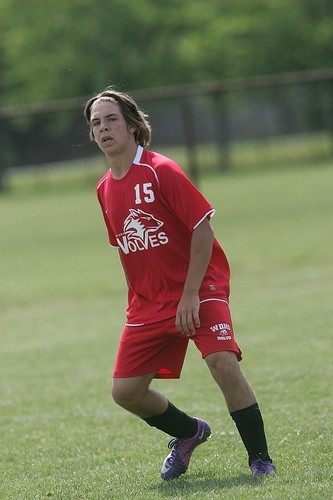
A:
83,83,275,481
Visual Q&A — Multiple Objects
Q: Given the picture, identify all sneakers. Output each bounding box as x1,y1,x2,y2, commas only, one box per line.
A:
160,417,211,481
250,458,276,480
